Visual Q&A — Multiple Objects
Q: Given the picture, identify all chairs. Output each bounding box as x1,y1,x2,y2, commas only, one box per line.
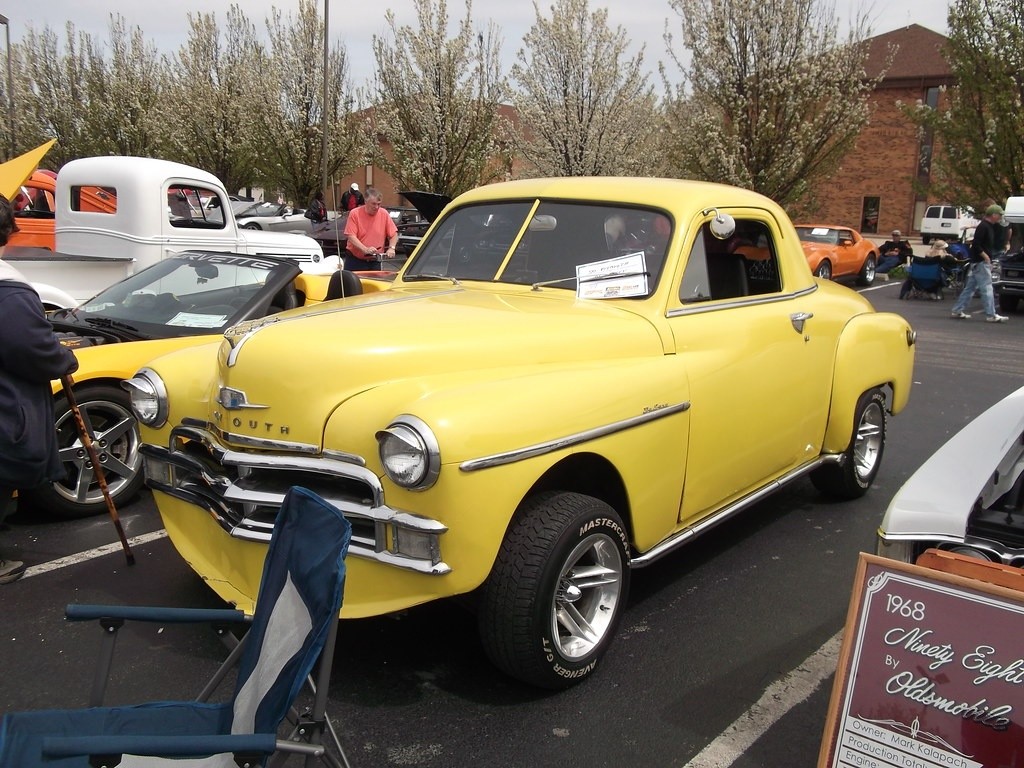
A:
257,271,297,316
323,270,362,302
704,254,750,302
0,485,350,768
909,255,943,302
947,257,972,299
878,241,910,276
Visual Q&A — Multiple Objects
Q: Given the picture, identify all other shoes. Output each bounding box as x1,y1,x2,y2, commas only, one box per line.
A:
986,314,1009,322
0,559,26,585
952,311,971,318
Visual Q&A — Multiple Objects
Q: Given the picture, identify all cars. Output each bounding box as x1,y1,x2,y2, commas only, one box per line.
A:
7,168,531,277
124,176,918,688
4,153,345,323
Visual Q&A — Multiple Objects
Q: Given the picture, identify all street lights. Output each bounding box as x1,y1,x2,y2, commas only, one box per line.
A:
0,15,16,159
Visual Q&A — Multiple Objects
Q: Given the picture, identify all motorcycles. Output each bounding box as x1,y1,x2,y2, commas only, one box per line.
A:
27,250,396,517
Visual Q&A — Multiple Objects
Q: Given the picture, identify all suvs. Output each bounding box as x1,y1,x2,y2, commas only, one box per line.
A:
921,204,982,246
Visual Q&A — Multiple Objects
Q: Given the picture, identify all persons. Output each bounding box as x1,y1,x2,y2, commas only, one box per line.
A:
0,194,79,586
926,240,951,258
311,190,327,230
875,230,913,272
340,183,365,211
990,202,1012,282
951,205,1009,323
343,188,399,272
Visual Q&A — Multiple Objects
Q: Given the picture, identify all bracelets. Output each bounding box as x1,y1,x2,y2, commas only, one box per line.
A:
390,246,395,250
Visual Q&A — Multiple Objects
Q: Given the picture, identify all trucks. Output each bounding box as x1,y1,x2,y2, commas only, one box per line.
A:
993,196,1024,311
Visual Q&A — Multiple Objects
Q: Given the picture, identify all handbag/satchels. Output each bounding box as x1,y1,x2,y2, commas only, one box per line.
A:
304,208,320,223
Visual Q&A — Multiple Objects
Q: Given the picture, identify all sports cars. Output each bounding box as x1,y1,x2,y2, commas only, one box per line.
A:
734,223,881,284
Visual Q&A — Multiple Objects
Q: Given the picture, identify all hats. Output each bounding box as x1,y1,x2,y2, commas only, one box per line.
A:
986,205,1005,215
893,230,900,235
351,183,358,190
933,240,948,249
